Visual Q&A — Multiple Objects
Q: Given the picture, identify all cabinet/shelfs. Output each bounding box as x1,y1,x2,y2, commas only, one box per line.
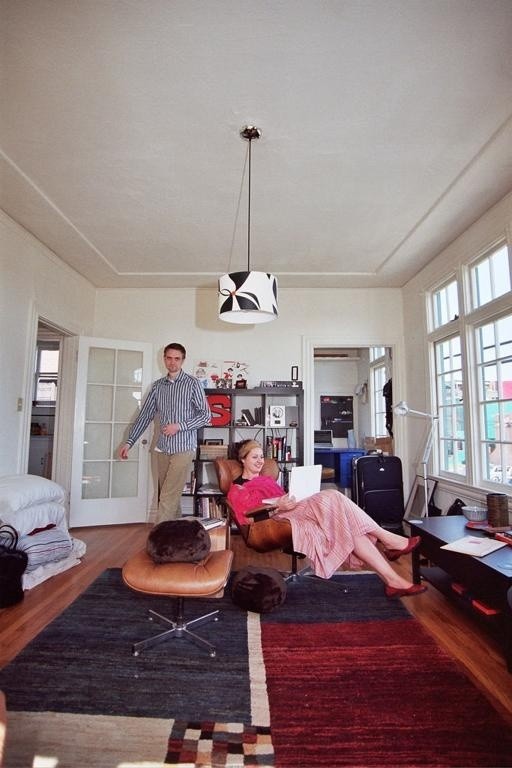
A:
200,526,231,553
27,433,52,479
180,388,305,535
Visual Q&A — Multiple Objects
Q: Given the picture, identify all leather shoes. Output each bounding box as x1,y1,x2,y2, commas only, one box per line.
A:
382,536,421,562
385,580,426,598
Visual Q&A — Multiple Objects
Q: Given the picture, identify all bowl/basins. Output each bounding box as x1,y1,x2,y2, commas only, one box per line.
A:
461,505,487,522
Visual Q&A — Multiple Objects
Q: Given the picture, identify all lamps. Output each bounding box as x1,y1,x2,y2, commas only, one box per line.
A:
389,396,441,518
216,125,278,327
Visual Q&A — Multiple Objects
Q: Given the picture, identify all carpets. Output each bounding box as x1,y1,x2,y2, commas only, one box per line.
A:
0,568,512,768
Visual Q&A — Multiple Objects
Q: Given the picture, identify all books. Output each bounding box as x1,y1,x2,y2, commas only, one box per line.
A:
197,496,224,519
197,495,227,530
440,536,508,557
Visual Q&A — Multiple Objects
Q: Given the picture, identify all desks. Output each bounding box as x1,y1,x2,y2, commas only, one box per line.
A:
315,448,365,488
399,514,510,674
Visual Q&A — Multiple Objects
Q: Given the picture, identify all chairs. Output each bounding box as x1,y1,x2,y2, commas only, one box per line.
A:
212,454,346,597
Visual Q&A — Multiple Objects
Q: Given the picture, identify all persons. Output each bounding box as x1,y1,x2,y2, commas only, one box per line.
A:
226,439,427,599
118,343,212,528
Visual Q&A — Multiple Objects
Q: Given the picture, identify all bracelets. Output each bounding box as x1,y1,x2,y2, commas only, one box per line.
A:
271,509,278,514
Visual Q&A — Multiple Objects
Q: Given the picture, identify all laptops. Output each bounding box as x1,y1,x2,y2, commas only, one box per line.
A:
314,429,334,448
262,464,324,505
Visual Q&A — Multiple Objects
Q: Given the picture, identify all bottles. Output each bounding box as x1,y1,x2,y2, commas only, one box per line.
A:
347,430,356,449
266,436,273,460
41,423,48,436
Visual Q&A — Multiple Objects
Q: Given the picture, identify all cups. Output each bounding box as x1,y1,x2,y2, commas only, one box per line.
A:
487,493,508,528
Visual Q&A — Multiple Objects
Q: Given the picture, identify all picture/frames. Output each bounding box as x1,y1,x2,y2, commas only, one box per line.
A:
400,476,437,520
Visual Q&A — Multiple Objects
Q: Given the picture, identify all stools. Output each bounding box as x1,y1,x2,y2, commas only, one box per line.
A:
121,542,236,660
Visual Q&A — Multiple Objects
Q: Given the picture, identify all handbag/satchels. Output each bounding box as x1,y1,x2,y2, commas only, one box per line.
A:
0,525,28,609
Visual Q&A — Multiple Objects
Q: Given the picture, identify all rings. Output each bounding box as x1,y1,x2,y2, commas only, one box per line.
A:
165,427,166,430
